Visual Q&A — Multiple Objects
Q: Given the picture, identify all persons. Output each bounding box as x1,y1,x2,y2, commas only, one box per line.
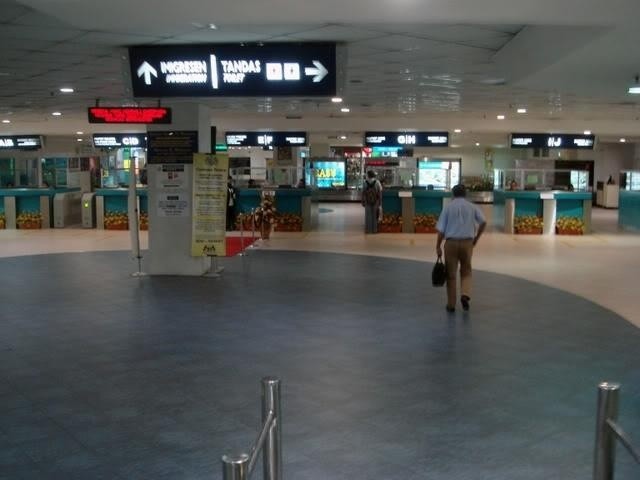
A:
7,182,12,187
140,163,147,184
296,179,305,189
567,184,573,191
226,175,237,231
42,181,48,188
432,185,486,311
510,180,519,191
247,179,256,188
361,170,383,233
91,166,100,189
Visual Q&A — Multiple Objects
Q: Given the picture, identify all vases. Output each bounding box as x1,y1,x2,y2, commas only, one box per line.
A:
105,224,148,230
378,225,437,233
237,223,301,232
0,224,41,229
517,227,582,234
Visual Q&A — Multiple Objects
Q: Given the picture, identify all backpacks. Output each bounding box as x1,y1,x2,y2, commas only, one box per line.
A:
365,180,378,205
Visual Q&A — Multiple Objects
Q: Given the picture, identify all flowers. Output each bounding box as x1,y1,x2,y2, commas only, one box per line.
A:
104,209,148,225
378,215,436,226
514,216,586,231
0,213,41,224
236,213,303,224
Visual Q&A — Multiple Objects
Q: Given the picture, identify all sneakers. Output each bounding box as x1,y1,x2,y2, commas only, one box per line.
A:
460,296,470,311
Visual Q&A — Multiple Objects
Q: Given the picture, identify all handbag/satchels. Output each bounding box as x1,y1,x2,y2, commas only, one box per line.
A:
432,262,446,286
